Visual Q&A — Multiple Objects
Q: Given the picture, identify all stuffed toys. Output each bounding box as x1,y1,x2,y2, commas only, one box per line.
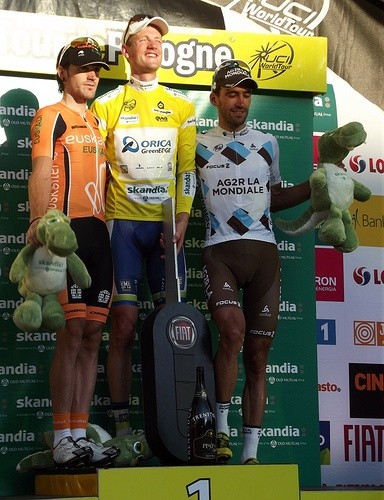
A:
275,122,372,253
9,210,91,331
16,423,151,473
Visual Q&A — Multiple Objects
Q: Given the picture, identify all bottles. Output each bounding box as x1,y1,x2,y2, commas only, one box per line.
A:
188,364,218,466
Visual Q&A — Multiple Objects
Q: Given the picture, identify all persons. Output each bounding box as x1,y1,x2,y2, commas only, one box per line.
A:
106,61,347,465
25,38,121,468
90,14,197,436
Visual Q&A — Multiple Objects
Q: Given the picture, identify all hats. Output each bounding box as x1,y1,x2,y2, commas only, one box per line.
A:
122,17,169,44
57,46,111,71
212,59,258,93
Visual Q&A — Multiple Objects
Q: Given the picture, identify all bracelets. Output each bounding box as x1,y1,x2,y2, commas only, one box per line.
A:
29,216,42,224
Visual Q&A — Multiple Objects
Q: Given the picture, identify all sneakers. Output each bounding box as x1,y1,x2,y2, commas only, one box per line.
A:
74,437,119,466
53,437,96,474
216,433,233,459
242,458,260,465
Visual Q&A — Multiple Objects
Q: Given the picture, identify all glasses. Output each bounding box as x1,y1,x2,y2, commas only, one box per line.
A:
58,36,101,66
213,60,252,85
124,14,155,43
